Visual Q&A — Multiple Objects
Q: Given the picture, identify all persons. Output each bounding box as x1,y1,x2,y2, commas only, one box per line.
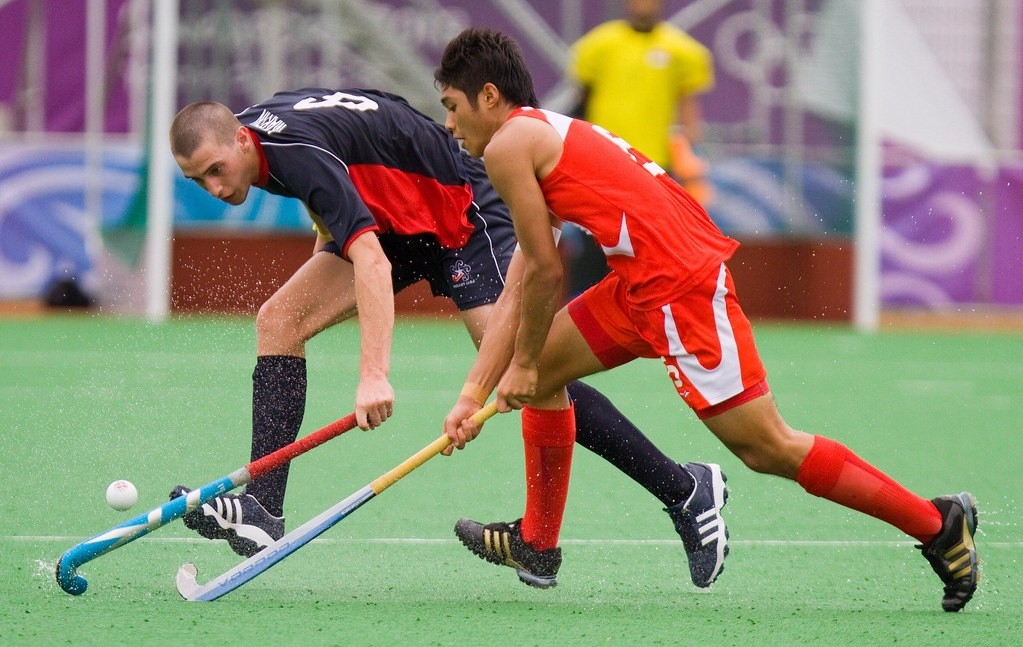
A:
170,86,730,589
432,25,980,615
539,0,713,314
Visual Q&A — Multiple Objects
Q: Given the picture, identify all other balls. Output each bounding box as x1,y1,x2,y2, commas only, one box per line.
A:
104,477,141,514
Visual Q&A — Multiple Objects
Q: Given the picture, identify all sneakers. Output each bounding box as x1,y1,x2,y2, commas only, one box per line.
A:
453,518,563,590
914,491,980,611
661,462,729,589
168,484,285,559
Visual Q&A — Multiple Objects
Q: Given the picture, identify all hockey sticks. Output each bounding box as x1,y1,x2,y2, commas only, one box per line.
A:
173,393,499,603
53,408,372,597
